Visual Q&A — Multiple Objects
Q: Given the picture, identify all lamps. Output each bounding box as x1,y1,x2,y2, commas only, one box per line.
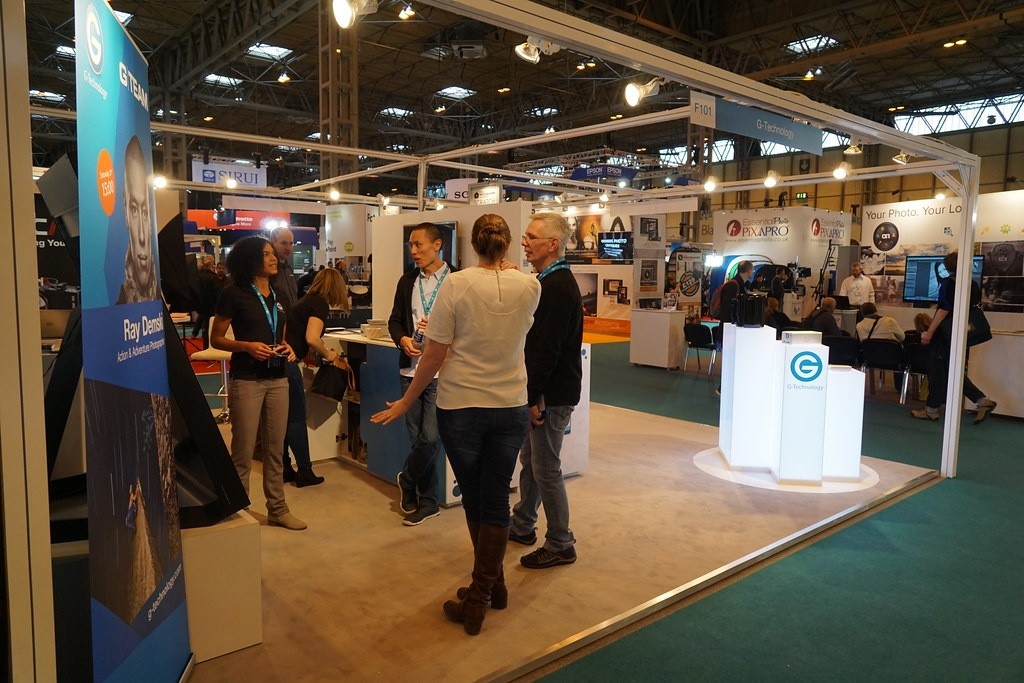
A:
936,187,953,200
600,190,613,203
198,145,212,165
832,162,853,180
251,45,262,169
203,109,214,122
763,169,783,188
575,55,596,70
892,150,911,165
624,75,671,109
332,0,378,28
705,175,720,193
278,66,291,83
555,192,568,204
844,135,863,155
235,94,245,102
397,2,416,20
514,36,562,67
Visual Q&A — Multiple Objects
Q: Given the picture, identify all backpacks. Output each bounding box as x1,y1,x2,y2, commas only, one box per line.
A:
797,309,827,333
710,276,742,320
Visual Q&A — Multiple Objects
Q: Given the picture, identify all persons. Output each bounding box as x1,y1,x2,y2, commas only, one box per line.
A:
368,213,541,638
211,235,307,531
113,135,161,306
387,223,462,526
279,269,352,488
665,274,680,297
310,260,348,284
506,210,584,571
718,252,997,425
194,247,226,339
251,225,297,462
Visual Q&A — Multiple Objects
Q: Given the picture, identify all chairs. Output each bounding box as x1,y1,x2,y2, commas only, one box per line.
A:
900,338,937,404
708,326,724,375
192,315,237,423
862,336,922,394
820,334,864,367
683,323,715,376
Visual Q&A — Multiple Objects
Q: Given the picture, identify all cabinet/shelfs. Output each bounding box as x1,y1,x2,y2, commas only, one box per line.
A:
282,339,445,505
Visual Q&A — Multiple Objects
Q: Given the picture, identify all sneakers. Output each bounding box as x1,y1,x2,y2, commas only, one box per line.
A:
911,405,940,421
396,471,419,515
519,543,577,568
402,502,441,528
973,397,998,425
507,525,538,547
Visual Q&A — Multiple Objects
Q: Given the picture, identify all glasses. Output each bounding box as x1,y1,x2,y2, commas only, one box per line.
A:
521,234,555,243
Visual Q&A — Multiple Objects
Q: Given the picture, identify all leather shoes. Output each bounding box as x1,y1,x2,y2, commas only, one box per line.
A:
266,512,308,532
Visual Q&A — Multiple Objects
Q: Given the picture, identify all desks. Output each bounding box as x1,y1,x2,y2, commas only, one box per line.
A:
629,307,686,370
280,325,593,509
960,328,1024,420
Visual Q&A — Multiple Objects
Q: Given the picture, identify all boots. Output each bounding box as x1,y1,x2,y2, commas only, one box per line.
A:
443,520,512,638
293,461,326,488
455,515,510,611
281,456,298,484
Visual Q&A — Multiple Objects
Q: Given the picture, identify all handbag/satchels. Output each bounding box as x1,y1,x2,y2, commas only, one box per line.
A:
310,347,357,404
941,276,993,350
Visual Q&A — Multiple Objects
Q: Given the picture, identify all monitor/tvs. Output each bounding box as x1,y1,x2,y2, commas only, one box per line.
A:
597,231,633,264
904,257,984,305
